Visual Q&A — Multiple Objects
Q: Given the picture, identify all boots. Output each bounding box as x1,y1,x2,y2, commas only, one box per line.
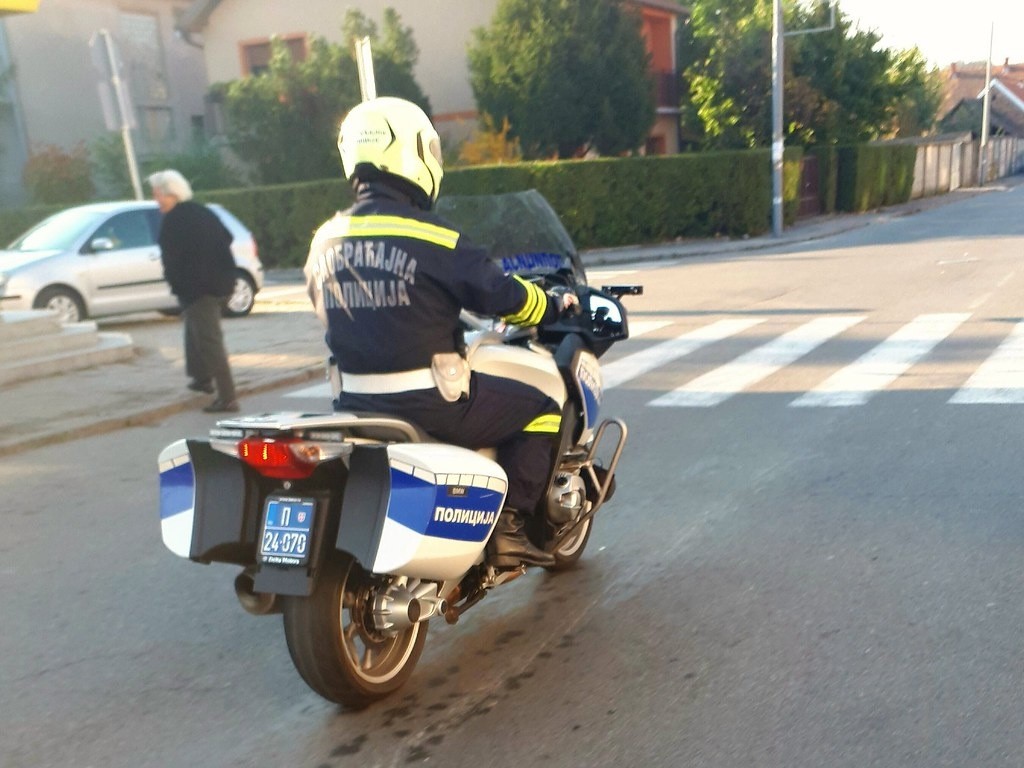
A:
487,507,557,570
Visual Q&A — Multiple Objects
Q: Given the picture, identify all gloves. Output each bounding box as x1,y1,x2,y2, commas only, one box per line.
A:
551,286,583,321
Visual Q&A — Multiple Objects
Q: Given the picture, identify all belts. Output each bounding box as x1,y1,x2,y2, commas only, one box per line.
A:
341,369,433,394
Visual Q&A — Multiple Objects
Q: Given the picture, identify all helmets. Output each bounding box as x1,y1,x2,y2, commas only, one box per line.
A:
337,96,444,211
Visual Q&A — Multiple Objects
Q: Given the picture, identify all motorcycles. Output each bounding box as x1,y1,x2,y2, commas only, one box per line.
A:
157,190,645,709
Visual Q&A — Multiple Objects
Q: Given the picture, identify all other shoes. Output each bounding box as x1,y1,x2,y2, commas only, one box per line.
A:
205,398,241,412
188,376,214,394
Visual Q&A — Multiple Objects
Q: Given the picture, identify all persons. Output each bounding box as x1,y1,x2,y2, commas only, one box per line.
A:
147,166,243,415
300,93,583,570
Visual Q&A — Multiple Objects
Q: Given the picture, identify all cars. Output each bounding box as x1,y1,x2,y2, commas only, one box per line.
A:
0,199,264,323
